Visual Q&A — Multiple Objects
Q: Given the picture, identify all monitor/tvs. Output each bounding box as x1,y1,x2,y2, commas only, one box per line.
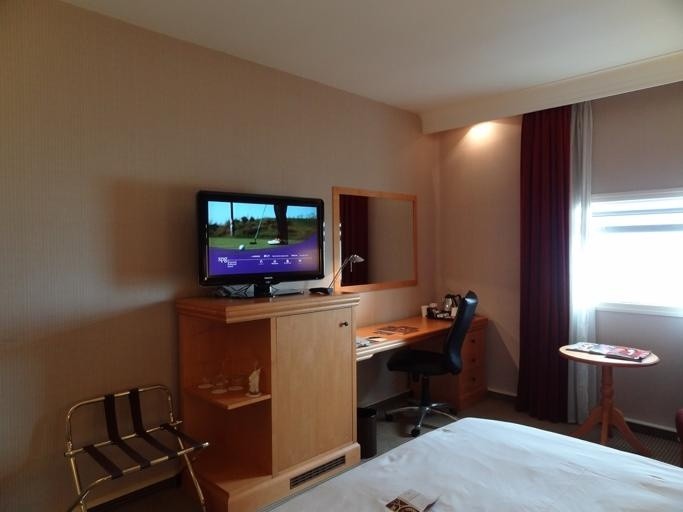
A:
195,190,326,299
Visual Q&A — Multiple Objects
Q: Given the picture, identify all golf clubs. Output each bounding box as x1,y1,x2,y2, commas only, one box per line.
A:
249,204,267,245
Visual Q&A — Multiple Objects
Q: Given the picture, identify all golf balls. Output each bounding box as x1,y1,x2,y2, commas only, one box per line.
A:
238,244,245,250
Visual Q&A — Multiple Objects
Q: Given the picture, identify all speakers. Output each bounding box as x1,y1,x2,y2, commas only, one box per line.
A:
357,407,377,458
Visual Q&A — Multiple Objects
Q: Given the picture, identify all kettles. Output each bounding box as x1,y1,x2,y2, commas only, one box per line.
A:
440,293,463,313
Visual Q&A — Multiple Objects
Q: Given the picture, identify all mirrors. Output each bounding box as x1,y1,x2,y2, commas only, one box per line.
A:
330,185,419,294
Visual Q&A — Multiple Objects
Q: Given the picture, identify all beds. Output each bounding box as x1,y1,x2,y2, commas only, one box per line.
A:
267,415,683,512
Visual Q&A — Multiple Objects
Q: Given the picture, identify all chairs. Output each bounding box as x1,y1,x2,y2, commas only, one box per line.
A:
377,290,480,437
61,383,210,512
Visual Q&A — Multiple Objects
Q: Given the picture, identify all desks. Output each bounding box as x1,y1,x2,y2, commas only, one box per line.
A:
357,310,487,416
559,343,661,456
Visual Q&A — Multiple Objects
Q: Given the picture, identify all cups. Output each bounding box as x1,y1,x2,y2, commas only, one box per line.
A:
421,303,438,318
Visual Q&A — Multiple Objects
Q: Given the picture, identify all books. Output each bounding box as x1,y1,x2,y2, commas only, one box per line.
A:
565,341,652,362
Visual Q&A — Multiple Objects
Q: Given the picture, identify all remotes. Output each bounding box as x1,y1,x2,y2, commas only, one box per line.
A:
309,286,333,295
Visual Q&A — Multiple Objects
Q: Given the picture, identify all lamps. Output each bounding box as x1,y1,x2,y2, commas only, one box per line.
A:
328,251,364,291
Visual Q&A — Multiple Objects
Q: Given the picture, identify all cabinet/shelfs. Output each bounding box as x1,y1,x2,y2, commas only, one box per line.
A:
174,294,363,512
